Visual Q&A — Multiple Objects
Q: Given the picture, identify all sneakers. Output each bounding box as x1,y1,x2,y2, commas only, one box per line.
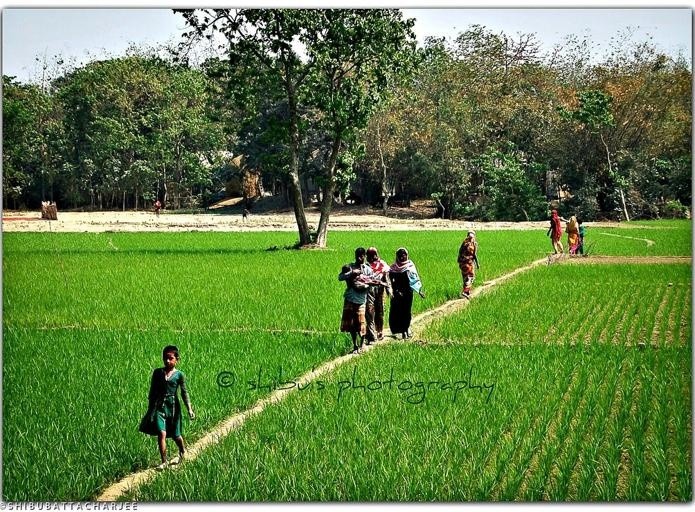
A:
351,333,383,354
178,453,185,462
402,328,412,338
462,293,469,299
157,460,169,469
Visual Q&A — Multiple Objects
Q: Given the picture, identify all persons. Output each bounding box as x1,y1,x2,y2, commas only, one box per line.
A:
385,247,427,341
152,198,161,218
149,344,196,470
242,208,250,217
337,247,377,354
341,264,390,291
564,215,580,255
364,247,395,346
457,230,480,301
575,217,586,256
547,209,564,254
